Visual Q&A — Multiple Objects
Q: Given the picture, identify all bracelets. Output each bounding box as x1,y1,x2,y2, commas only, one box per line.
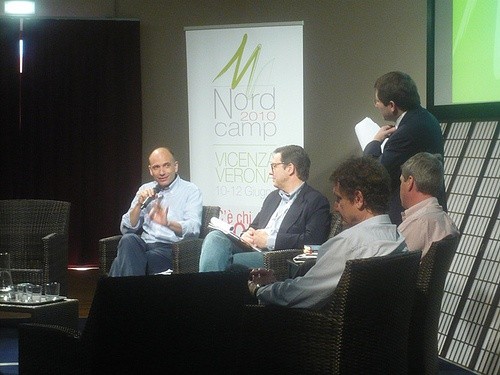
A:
252,279,275,295
167,220,170,228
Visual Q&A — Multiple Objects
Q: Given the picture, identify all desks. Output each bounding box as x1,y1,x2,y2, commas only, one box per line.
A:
0,298,81,367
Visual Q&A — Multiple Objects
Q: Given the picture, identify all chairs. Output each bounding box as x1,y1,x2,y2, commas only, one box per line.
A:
0,199,460,375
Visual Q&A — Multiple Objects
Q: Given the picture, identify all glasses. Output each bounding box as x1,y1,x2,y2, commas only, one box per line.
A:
270,163,285,169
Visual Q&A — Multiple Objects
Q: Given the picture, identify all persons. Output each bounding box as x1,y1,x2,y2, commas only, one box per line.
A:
199,145,329,272
362,71,447,227
397,152,458,264
107,147,203,277
248,156,408,307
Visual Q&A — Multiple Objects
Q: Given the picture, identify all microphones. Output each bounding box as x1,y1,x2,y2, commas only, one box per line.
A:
140,185,161,209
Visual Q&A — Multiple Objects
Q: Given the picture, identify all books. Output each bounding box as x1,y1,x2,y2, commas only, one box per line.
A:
207,216,265,253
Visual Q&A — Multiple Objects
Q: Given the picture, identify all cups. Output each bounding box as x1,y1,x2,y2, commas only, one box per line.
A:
13,281,60,302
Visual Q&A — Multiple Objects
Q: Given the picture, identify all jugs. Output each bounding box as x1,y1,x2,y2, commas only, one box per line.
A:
0,252,13,302
